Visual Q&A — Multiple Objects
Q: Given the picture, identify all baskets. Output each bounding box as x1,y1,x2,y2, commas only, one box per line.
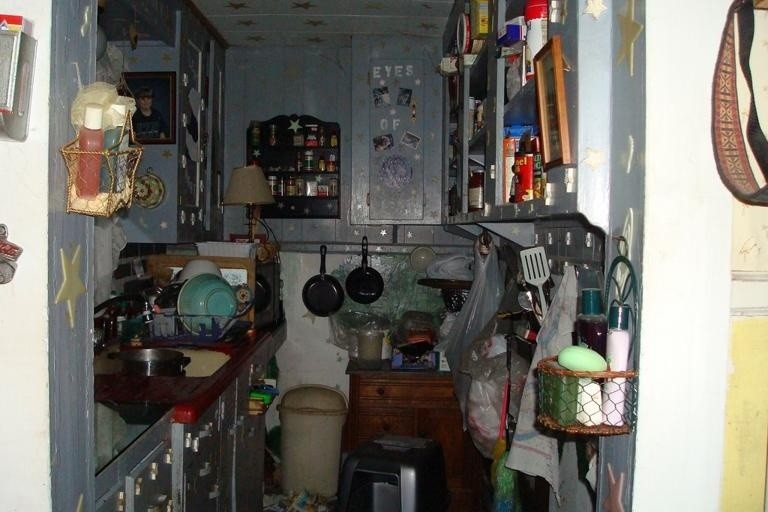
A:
61,110,143,219
536,237,637,435
145,311,237,340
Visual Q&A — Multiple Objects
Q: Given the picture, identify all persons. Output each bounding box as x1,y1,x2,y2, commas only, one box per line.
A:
131,85,165,140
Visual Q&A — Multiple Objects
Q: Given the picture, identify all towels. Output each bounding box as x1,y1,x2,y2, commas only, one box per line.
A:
505,263,578,508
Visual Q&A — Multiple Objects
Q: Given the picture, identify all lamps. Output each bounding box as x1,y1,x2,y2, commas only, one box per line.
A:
221,165,276,244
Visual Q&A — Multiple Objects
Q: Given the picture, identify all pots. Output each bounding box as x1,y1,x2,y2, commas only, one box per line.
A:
108,347,192,374
303,245,345,318
346,236,384,305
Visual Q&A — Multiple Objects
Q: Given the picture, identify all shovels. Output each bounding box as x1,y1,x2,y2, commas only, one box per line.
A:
520,246,550,321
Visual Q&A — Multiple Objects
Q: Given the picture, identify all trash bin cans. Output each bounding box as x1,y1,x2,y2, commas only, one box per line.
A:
277,383,349,499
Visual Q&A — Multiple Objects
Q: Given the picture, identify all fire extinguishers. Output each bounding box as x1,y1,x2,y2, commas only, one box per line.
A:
524,0,549,82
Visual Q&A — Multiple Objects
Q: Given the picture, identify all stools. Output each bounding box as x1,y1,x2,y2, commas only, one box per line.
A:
338,435,452,511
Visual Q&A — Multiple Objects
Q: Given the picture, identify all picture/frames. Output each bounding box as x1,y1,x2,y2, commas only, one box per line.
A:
120,71,176,145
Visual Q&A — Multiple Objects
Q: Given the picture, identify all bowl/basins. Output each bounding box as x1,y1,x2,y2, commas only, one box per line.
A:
171,258,221,287
178,273,237,338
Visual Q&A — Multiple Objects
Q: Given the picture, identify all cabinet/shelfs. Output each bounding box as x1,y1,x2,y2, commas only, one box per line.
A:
91,1,229,243
443,0,608,234
344,359,483,511
92,407,172,512
351,33,441,225
171,356,264,512
246,113,340,219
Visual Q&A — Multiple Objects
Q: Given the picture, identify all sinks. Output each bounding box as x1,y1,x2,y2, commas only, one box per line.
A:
121,344,232,382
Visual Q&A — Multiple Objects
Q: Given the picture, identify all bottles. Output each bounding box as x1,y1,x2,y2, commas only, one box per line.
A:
104,287,152,340
576,288,608,354
101,105,133,194
248,121,338,197
603,305,629,427
76,104,105,199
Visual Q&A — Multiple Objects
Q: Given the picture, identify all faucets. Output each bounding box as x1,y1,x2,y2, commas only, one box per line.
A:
95,293,147,318
